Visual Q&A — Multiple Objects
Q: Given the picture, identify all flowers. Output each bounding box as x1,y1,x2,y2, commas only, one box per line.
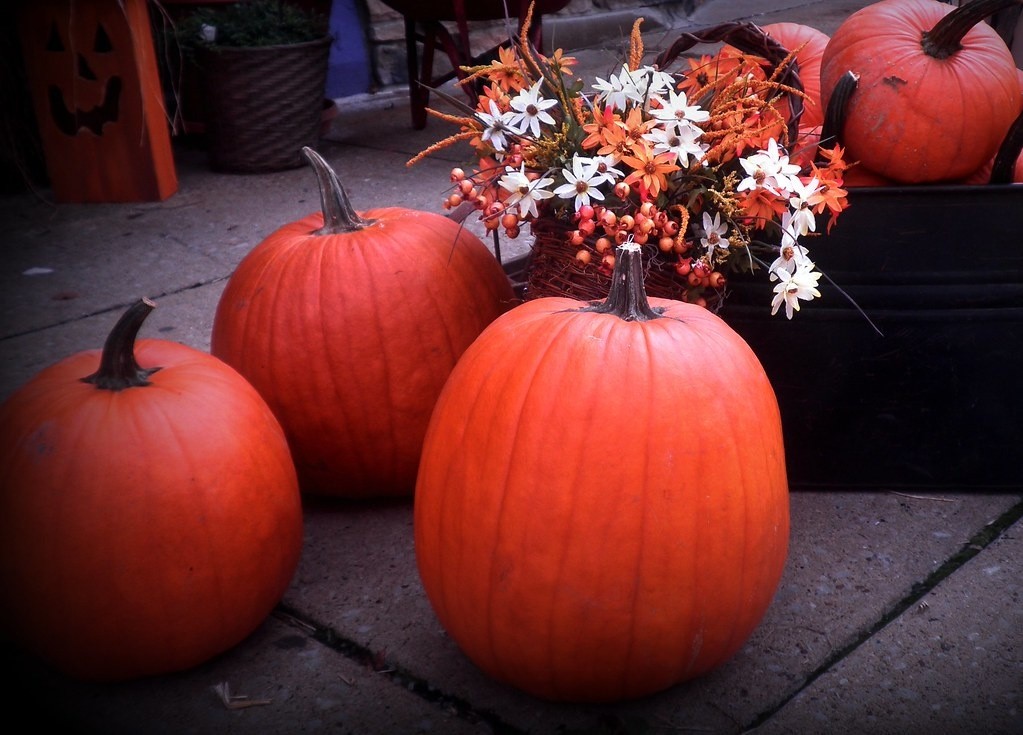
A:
402,1,859,320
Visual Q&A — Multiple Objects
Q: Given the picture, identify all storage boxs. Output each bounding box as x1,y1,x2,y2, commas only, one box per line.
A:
519,217,730,315
713,182,1021,499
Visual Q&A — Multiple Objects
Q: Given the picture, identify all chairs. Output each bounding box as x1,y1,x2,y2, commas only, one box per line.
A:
380,0,571,132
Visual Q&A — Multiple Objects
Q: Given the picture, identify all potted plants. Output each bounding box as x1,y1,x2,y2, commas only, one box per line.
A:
172,1,334,177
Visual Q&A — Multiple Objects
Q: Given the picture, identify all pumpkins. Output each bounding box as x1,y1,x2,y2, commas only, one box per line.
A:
0,0,1023,704
